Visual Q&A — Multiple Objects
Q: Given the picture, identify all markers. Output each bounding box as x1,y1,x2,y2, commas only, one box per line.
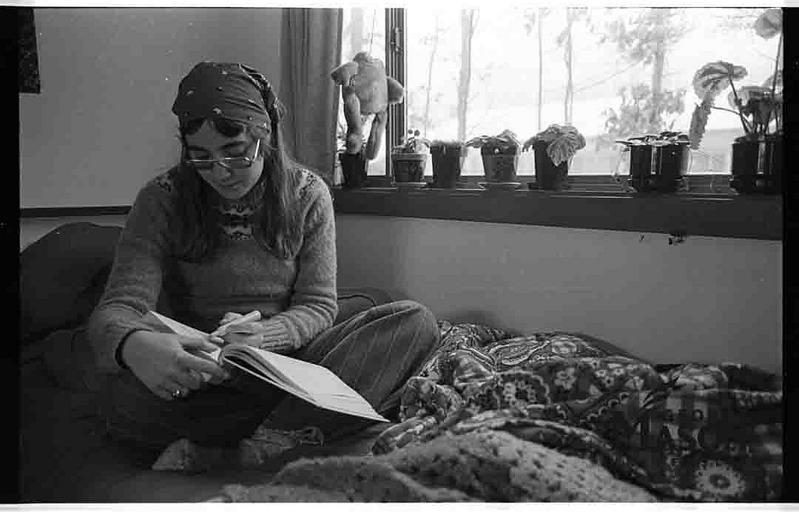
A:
210,310,261,337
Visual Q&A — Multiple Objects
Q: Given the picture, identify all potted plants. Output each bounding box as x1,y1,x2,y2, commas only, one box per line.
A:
333,124,586,189
614,9,783,196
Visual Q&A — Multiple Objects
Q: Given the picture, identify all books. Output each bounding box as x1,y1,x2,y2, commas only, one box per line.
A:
140,309,392,425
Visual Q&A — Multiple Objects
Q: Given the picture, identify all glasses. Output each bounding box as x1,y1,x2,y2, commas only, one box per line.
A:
179,138,261,169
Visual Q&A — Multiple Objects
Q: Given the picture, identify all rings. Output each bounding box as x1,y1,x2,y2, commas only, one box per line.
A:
171,390,180,397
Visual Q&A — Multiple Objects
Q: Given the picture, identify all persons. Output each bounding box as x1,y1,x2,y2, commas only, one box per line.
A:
42,60,440,473
